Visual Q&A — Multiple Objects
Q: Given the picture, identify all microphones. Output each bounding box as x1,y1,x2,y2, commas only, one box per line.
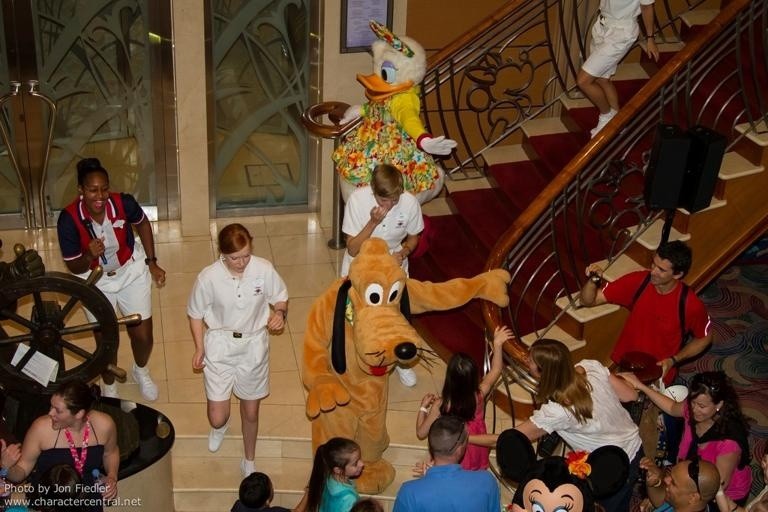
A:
82,217,106,263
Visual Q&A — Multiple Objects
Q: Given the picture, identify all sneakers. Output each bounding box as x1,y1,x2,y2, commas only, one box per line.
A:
132,363,157,402
208,420,231,453
396,365,418,387
590,108,618,136
103,382,118,398
239,456,256,478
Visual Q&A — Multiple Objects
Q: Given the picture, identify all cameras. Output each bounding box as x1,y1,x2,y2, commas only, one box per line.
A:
636,469,646,491
589,271,603,287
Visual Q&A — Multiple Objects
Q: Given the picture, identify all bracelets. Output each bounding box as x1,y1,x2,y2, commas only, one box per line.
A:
645,34,655,39
398,245,412,259
667,355,676,367
274,308,287,319
143,257,157,265
419,406,428,413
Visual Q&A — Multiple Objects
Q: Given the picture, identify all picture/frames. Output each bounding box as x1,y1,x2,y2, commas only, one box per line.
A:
339,0,394,54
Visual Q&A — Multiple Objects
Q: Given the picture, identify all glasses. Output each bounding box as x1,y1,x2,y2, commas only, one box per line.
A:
687,454,701,494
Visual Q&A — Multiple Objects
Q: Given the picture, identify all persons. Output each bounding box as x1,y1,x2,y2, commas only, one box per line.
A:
186,224,288,478
338,164,424,279
414,326,514,472
7,376,119,512
350,498,384,512
308,437,364,511
393,336,768,512
579,240,712,386
0,439,32,512
227,470,311,512
6,460,88,511
56,157,165,404
574,0,660,139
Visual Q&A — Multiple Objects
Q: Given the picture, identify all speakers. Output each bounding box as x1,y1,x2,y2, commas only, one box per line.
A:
645,123,727,214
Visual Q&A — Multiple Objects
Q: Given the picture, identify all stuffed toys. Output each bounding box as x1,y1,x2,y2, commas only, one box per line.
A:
301,237,510,495
